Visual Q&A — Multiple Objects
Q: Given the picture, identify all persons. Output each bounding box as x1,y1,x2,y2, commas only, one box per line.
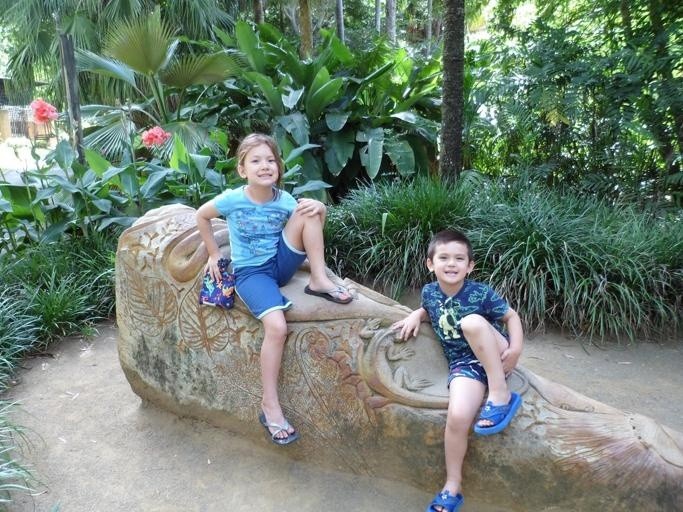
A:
195,133,354,445
391,230,525,512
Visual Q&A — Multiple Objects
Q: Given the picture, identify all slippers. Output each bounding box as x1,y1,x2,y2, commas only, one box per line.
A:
259,414,299,445
427,492,463,512
473,392,521,435
305,285,352,304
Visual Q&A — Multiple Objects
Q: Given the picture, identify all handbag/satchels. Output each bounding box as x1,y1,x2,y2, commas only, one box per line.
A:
200,259,236,308
